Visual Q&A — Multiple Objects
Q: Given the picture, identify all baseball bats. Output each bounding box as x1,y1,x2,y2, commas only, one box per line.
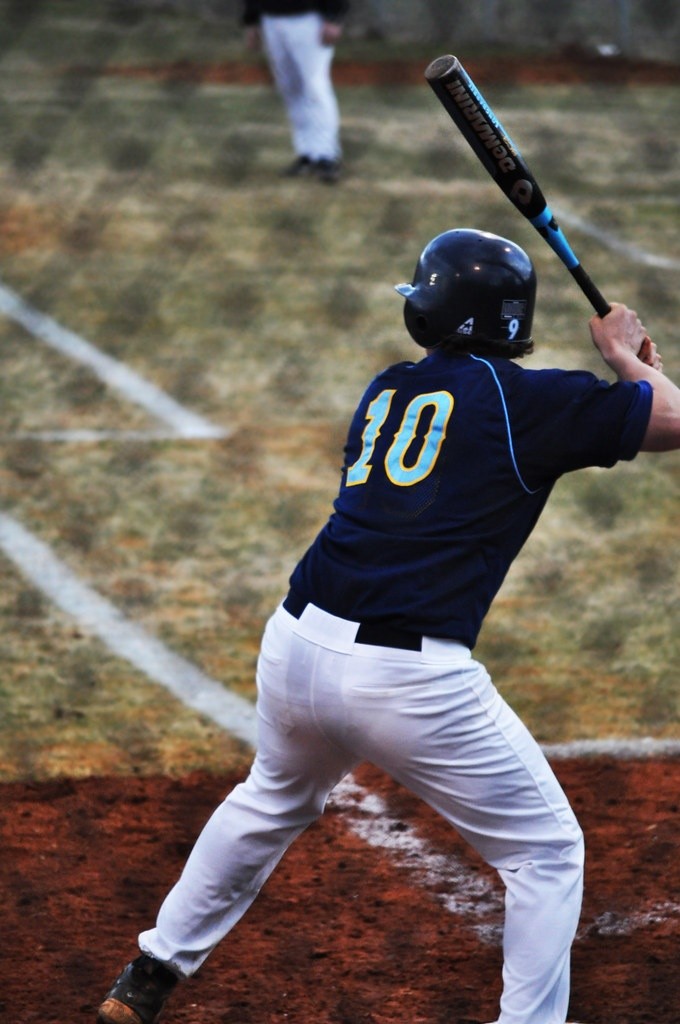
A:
422,51,656,367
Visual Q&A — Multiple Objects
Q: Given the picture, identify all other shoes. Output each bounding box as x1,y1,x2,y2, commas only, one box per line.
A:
98,955,181,1024
279,155,317,179
316,158,343,181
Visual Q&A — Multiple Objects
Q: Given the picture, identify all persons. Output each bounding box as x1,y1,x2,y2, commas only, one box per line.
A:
95,227,680,1024
243,0,352,185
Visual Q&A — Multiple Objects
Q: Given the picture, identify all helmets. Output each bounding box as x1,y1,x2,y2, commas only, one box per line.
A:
394,228,538,358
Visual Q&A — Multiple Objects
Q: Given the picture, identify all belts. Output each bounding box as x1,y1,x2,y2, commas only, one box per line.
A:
284,588,422,651
